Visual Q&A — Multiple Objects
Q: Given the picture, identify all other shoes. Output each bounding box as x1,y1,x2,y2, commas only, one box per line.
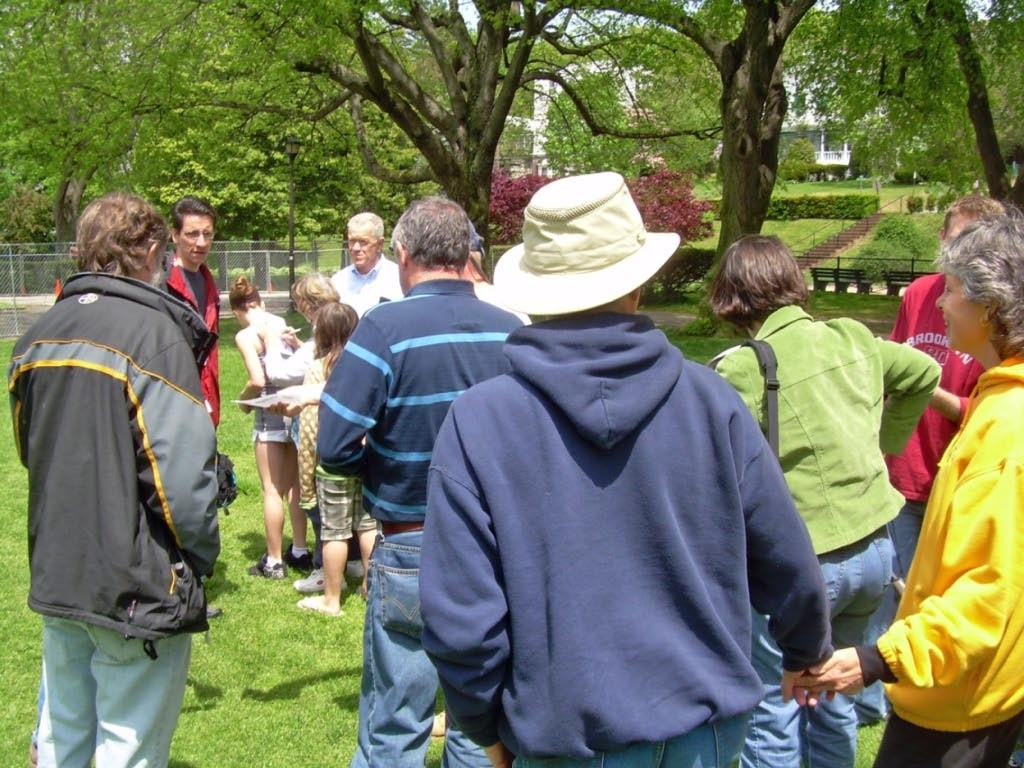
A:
298,594,344,617
431,710,448,738
205,605,223,618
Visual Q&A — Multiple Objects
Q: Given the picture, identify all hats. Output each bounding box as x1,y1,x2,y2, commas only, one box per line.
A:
493,172,682,316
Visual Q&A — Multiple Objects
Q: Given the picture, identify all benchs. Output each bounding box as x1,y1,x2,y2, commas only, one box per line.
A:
808,267,941,297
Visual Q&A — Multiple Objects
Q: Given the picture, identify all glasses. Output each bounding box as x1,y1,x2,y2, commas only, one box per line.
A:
185,228,218,242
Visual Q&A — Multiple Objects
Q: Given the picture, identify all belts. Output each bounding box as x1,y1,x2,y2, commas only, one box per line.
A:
381,518,426,537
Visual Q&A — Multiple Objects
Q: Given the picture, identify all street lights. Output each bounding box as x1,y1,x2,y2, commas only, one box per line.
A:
284,133,301,312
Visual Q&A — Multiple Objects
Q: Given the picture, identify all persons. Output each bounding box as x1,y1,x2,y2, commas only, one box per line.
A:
226,209,542,626
878,195,1024,585
794,212,1024,768
11,194,220,768
317,197,533,768
704,232,941,768
158,196,218,591
416,171,833,753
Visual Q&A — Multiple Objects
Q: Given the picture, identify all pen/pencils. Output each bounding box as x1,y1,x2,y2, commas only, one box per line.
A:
288,328,301,335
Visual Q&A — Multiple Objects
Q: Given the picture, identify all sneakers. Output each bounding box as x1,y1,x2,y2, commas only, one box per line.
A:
293,568,349,593
283,544,314,573
343,559,364,578
356,585,363,596
256,550,286,580
